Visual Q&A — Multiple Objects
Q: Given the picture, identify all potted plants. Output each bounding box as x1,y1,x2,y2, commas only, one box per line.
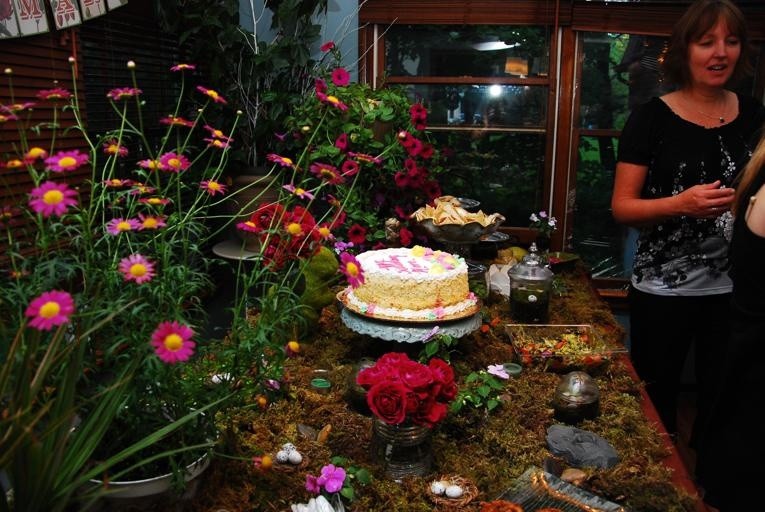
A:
158,0,333,249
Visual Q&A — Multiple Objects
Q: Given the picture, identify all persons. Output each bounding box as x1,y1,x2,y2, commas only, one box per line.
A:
697,135,764,511
608,1,765,444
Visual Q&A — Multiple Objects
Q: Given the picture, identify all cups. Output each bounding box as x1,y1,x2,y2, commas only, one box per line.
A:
468,264,494,322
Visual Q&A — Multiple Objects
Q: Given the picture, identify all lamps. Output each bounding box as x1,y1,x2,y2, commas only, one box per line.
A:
471,25,524,54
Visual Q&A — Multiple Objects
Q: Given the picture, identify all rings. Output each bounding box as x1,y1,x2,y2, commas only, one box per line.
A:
712,206,717,216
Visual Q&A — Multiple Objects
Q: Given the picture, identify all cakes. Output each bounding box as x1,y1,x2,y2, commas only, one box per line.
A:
343,245,478,320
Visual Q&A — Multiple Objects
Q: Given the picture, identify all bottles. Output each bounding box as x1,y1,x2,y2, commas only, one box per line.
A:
506,242,554,329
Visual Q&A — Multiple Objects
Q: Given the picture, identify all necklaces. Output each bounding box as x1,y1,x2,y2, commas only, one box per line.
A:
682,91,730,123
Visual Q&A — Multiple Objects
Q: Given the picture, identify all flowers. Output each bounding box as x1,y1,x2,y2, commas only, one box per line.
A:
356,351,462,424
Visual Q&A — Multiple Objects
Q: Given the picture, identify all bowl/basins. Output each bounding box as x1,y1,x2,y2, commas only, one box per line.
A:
455,198,480,211
415,206,506,241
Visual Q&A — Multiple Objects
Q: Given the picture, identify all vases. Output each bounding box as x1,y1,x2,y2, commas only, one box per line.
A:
368,419,433,479
